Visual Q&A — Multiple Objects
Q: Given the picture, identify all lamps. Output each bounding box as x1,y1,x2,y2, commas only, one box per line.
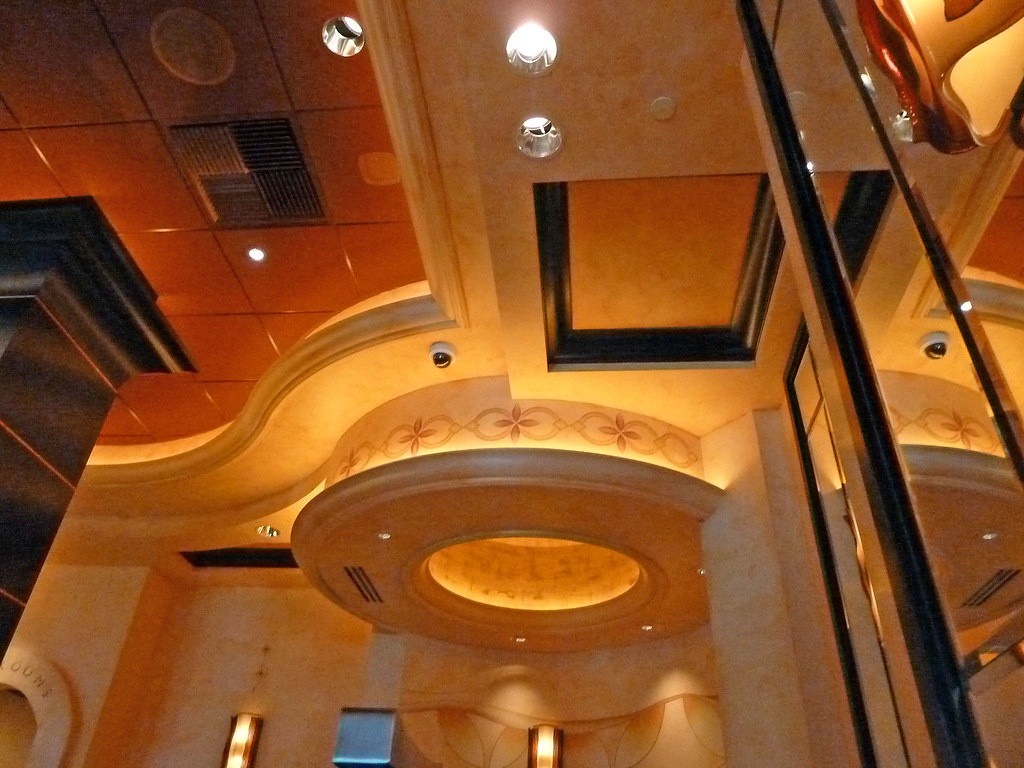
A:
220,712,263,768
527,724,564,768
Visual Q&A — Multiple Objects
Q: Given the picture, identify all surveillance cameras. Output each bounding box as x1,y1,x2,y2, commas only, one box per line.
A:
920,332,949,359
427,341,456,367
